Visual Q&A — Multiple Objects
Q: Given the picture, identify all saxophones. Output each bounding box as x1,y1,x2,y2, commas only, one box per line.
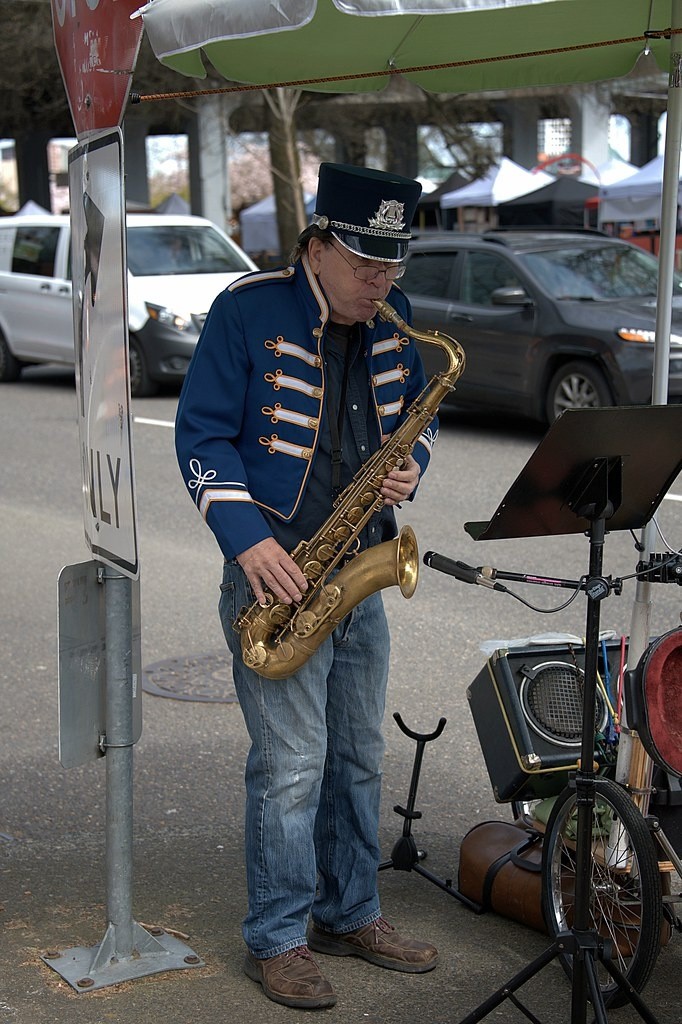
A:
231,298,466,681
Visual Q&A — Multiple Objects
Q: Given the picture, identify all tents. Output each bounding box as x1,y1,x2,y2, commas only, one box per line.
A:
15,157,680,254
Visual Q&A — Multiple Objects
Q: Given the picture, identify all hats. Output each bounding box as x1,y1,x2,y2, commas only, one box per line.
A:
313,162,422,263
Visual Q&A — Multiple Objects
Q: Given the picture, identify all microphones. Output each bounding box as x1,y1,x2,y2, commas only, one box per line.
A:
423,551,507,593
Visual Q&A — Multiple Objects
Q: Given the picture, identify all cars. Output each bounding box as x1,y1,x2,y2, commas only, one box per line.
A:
392,224,682,433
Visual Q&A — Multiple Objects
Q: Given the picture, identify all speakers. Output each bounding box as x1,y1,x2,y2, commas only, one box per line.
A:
466,637,659,803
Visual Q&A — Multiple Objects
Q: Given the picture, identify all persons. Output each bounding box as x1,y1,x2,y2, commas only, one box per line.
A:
165,239,184,273
173,161,441,1010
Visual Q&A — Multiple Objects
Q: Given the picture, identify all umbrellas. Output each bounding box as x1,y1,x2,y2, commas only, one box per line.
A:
128,0,681,866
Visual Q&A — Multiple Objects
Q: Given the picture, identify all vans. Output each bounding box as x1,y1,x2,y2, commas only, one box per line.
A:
0,214,259,400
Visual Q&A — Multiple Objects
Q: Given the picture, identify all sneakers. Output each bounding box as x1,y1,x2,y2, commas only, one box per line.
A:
306,916,440,973
244,945,336,1008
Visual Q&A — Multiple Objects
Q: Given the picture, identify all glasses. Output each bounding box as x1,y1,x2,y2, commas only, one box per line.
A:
327,239,406,281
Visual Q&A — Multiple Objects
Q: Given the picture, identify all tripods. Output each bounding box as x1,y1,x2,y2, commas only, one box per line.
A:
377,712,483,916
465,403,682,1024
